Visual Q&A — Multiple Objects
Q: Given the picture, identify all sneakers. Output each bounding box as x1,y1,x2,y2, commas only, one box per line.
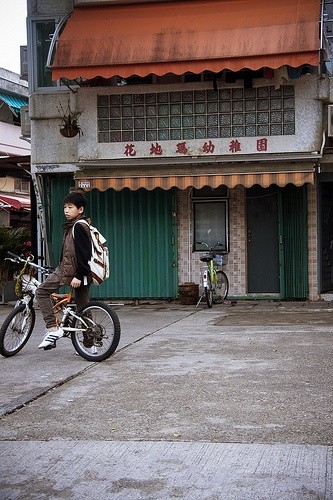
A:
75,342,98,357
37,325,64,349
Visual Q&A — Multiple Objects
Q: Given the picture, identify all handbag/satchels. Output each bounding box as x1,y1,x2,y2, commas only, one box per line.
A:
14,260,34,299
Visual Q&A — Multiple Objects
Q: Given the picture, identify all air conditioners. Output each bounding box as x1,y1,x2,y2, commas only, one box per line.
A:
20,106,31,135
20,46,27,82
327,104,333,137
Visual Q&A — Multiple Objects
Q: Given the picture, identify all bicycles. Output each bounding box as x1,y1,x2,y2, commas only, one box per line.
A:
195,241,229,309
0,251,121,362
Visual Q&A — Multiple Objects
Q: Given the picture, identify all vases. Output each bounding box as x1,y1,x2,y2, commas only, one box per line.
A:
178,282,199,305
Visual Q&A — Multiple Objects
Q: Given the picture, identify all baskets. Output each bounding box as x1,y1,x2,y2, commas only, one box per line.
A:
214,253,229,265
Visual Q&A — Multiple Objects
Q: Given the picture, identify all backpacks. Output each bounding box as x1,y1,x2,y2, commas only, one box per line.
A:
71,217,110,286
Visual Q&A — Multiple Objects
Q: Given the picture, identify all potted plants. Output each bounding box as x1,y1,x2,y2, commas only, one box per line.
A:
55,98,84,139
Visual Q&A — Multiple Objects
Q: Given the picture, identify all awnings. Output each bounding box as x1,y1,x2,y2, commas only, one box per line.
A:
0,194,31,210
0,93,29,112
52,0,319,80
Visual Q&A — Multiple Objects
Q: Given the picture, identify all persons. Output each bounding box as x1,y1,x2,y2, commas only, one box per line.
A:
35,191,98,356
